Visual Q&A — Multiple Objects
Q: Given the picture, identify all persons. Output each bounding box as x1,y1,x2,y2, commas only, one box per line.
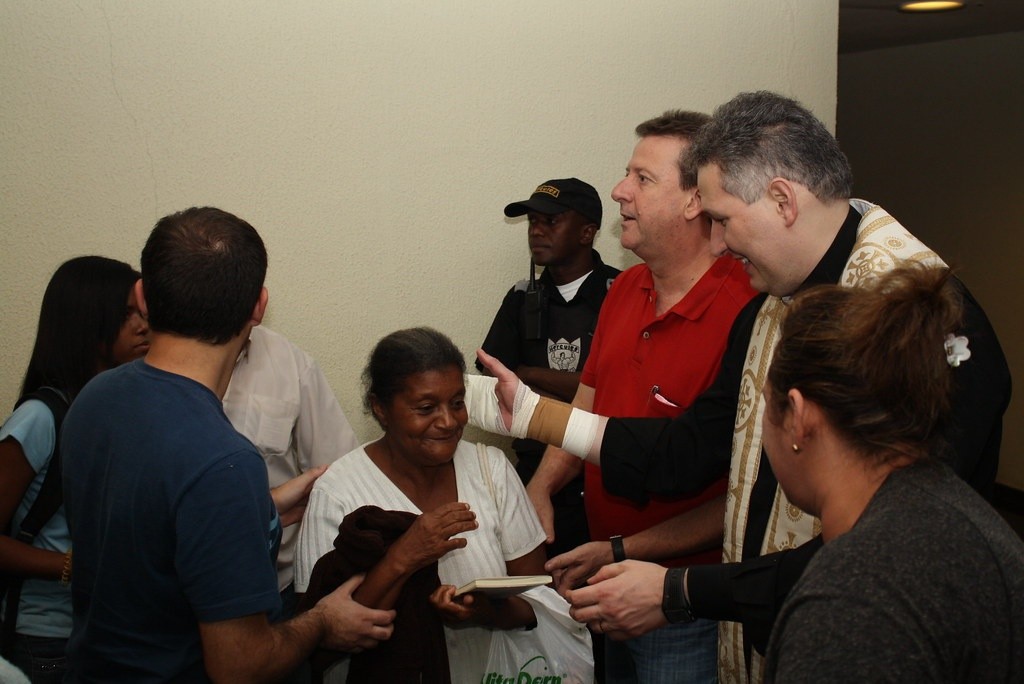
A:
223,326,361,623
0,255,147,683
61,208,397,684
524,110,762,684
761,271,1024,683
465,91,1012,684
294,328,549,684
475,177,621,555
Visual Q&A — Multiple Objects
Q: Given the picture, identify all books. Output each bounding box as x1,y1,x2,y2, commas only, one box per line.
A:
453,575,552,602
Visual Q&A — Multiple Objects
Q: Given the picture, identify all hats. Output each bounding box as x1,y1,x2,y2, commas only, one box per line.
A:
504,178,603,229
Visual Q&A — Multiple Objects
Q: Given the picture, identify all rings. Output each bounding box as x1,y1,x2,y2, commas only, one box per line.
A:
600,621,604,631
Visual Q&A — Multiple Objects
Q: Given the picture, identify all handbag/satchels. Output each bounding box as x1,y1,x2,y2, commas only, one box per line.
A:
481,585,596,683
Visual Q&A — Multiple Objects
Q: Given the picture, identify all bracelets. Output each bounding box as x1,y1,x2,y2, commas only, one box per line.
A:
62,548,72,586
663,567,690,624
610,535,625,562
